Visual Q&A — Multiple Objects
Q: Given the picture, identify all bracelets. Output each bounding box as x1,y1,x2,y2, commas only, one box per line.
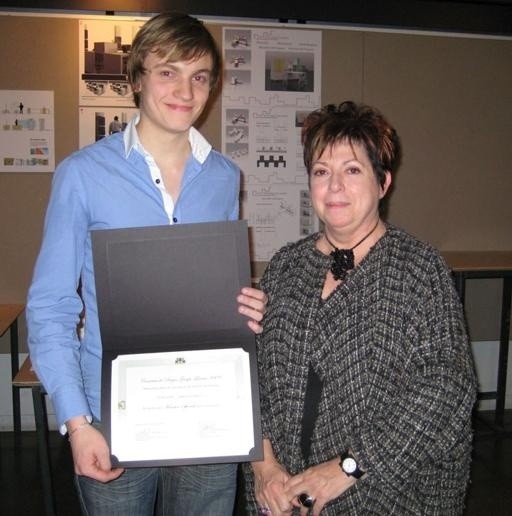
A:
68,422,89,443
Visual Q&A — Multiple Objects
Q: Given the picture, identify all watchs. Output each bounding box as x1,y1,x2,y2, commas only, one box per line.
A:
338,450,364,481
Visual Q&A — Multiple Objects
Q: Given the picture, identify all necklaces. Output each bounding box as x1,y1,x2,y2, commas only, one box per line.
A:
318,217,384,282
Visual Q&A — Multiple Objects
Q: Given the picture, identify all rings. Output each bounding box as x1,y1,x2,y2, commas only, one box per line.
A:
299,494,313,509
258,506,271,514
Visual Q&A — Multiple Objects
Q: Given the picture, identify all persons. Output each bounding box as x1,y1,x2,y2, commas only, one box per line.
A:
108,115,123,136
24,11,269,516
240,96,480,516
